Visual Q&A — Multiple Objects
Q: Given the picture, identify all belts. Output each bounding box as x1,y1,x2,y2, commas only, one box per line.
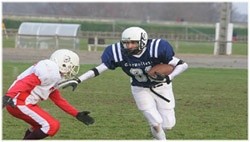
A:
131,81,170,102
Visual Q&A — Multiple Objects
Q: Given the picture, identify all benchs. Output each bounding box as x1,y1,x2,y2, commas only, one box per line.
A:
88,44,109,53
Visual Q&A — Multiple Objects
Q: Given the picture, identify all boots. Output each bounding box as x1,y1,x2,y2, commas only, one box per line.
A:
22,128,48,140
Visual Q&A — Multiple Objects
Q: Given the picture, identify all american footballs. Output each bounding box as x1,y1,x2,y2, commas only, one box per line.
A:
148,63,175,76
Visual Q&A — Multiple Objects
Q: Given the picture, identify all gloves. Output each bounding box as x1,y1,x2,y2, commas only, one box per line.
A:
76,110,95,125
57,76,81,91
2,95,14,108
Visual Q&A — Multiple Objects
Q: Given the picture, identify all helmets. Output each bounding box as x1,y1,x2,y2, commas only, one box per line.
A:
121,27,148,51
49,49,80,80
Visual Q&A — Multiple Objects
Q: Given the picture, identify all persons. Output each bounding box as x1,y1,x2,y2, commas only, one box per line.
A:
2,49,94,140
58,26,188,140
